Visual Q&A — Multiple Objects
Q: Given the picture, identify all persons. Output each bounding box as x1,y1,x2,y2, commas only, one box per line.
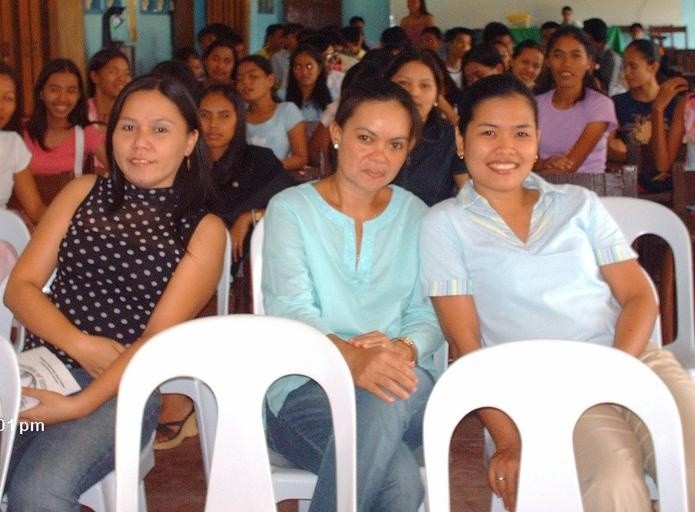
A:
235,55,308,172
0,75,229,509
0,65,52,226
416,25,442,55
146,62,199,93
191,24,243,53
526,27,618,199
78,49,135,129
268,26,298,103
480,20,519,58
650,75,695,222
284,49,336,184
379,25,406,40
379,36,411,61
581,16,625,99
506,38,545,90
192,81,292,280
382,48,465,206
558,5,584,28
256,77,450,510
610,38,674,213
22,59,112,211
199,38,237,93
537,20,558,48
298,14,370,77
457,46,504,91
250,22,283,64
173,48,203,81
434,28,475,116
396,0,436,48
629,22,644,39
418,75,694,512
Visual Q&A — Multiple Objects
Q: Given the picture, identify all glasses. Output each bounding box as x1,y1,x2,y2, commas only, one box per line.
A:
294,65,313,70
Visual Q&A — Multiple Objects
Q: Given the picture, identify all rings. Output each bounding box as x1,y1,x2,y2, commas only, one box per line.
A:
495,476,504,482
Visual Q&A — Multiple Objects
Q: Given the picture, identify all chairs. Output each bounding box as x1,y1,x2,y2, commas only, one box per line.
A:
599,196,692,365
2,210,43,357
78,228,231,507
250,209,318,510
423,338,689,511
113,313,356,511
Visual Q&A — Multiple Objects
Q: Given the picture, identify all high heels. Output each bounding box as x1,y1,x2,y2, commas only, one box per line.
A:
154,402,199,451
